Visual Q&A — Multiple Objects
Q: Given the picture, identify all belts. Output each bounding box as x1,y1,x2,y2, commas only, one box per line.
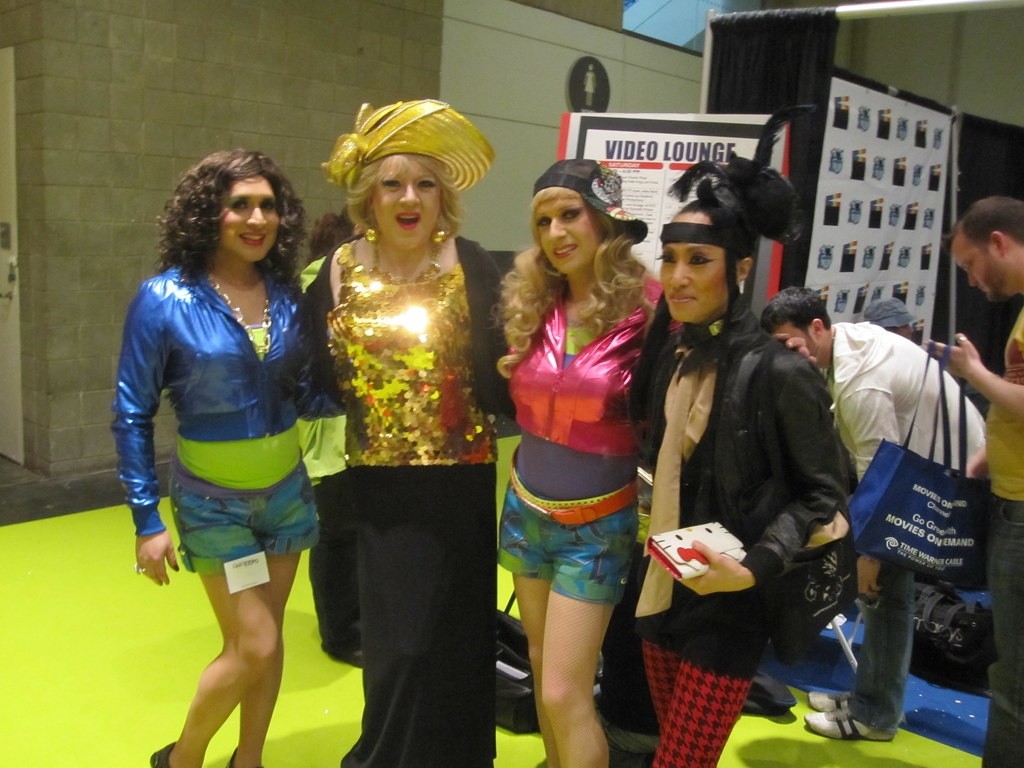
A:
510,443,640,526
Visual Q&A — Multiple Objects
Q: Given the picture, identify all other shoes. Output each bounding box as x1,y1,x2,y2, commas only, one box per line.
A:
150,743,174,768
227,748,238,767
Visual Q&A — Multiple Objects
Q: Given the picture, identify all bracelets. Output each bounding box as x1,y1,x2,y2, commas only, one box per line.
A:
863,554,879,562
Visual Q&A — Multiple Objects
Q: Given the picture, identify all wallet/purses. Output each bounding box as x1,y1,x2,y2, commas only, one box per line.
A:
647,521,752,580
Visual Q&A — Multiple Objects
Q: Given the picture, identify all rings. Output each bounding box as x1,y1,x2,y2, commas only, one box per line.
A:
132,564,148,576
958,335,967,342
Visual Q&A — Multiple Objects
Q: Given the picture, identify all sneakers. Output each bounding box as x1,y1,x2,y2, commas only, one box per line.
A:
805,691,898,741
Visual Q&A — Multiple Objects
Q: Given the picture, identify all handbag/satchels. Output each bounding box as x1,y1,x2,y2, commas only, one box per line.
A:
494,594,538,733
764,508,857,661
908,589,995,693
851,441,991,589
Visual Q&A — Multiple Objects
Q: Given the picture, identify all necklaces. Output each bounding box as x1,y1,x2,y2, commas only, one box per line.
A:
371,237,441,282
206,265,270,355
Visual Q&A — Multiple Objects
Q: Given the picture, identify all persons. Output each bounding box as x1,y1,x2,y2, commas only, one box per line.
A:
758,286,985,741
296,96,517,768
926,195,1023,768
496,156,819,767
111,147,347,768
628,158,842,767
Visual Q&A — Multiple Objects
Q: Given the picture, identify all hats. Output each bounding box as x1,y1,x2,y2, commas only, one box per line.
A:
318,98,492,192
533,161,649,245
864,297,916,328
